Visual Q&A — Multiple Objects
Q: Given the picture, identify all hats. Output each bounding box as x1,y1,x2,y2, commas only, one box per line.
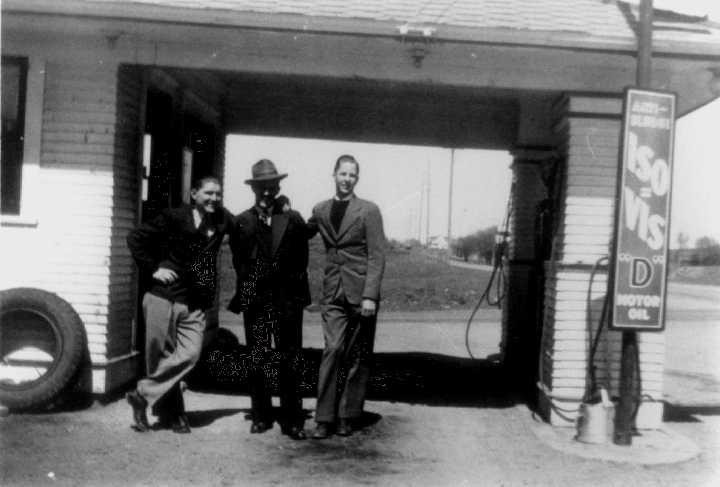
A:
243,158,287,186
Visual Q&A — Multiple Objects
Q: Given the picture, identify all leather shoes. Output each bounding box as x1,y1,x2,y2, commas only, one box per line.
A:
249,418,275,434
280,420,306,441
125,392,152,432
310,419,333,439
169,414,191,433
332,418,356,437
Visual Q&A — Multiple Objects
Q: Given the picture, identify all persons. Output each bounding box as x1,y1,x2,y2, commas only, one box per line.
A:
305,154,384,436
227,158,312,434
129,170,291,432
